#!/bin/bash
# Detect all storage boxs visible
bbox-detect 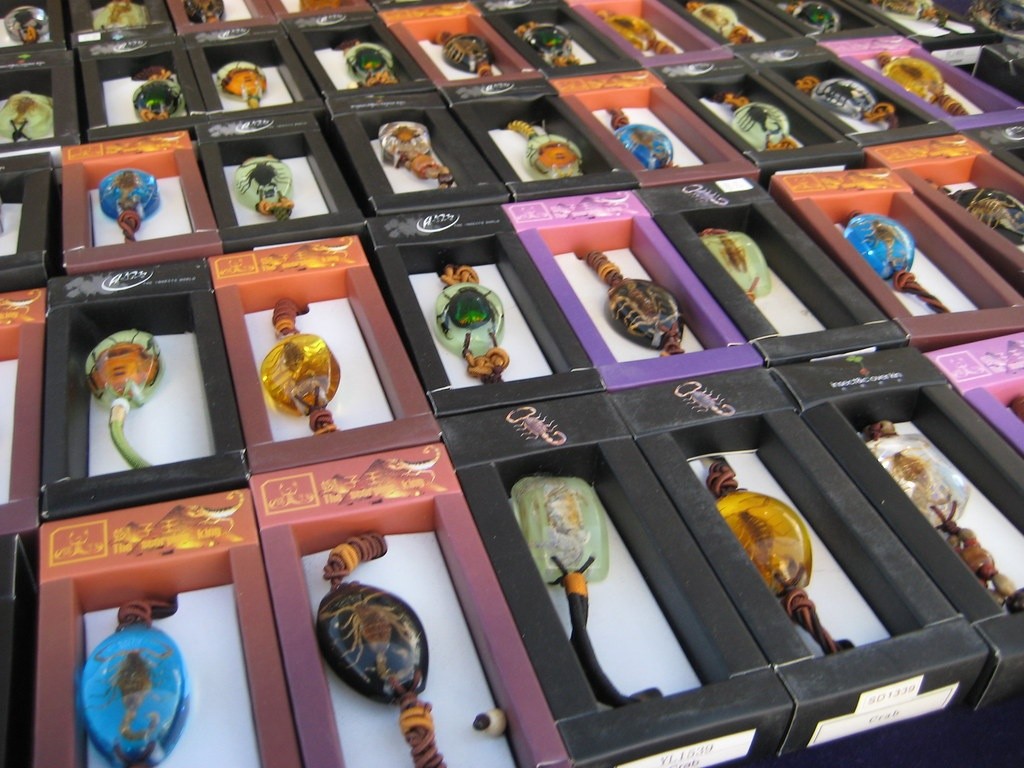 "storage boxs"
[0,0,1024,768]
